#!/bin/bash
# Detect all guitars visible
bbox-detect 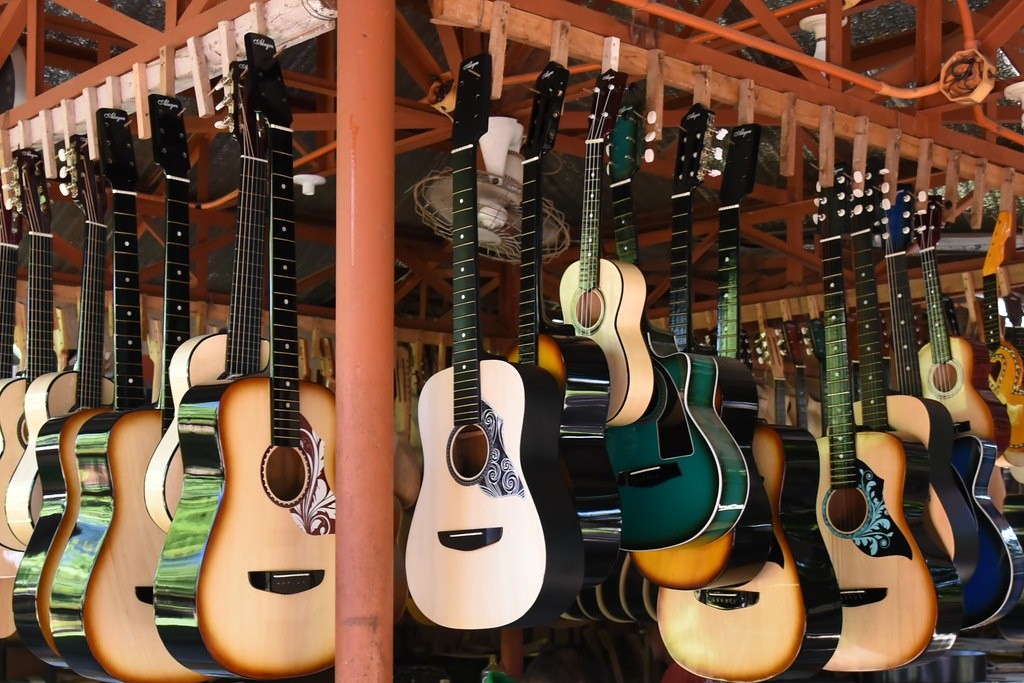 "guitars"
[153,33,339,682]
[557,64,656,428]
[402,50,590,635]
[603,101,1024,683]
[504,61,627,590]
[139,56,272,545]
[2,91,216,681]
